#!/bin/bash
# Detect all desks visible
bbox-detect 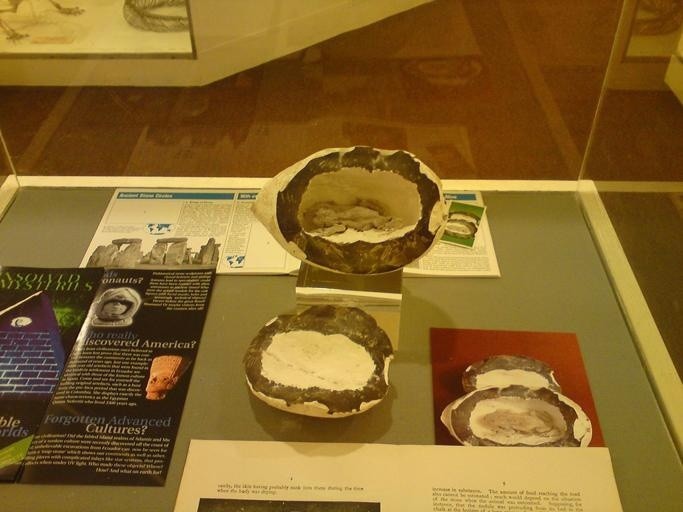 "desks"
[0,176,683,512]
